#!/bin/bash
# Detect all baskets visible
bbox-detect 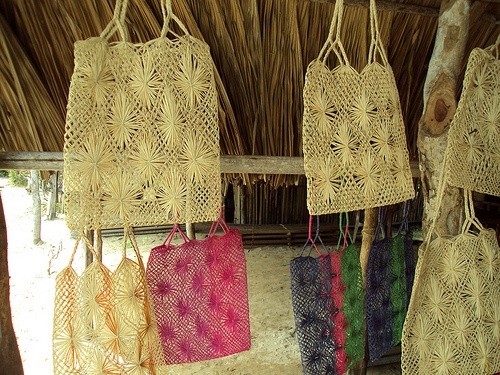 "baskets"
[442,30,500,199]
[145,222,252,365]
[300,0,415,216]
[62,0,223,231]
[399,145,500,374]
[357,201,418,364]
[51,232,168,375]
[289,214,367,374]
